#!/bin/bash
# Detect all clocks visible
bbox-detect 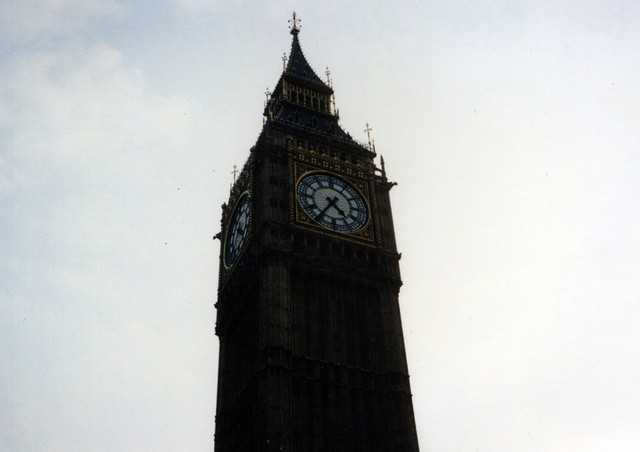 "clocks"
[221,189,253,270]
[295,167,372,236]
